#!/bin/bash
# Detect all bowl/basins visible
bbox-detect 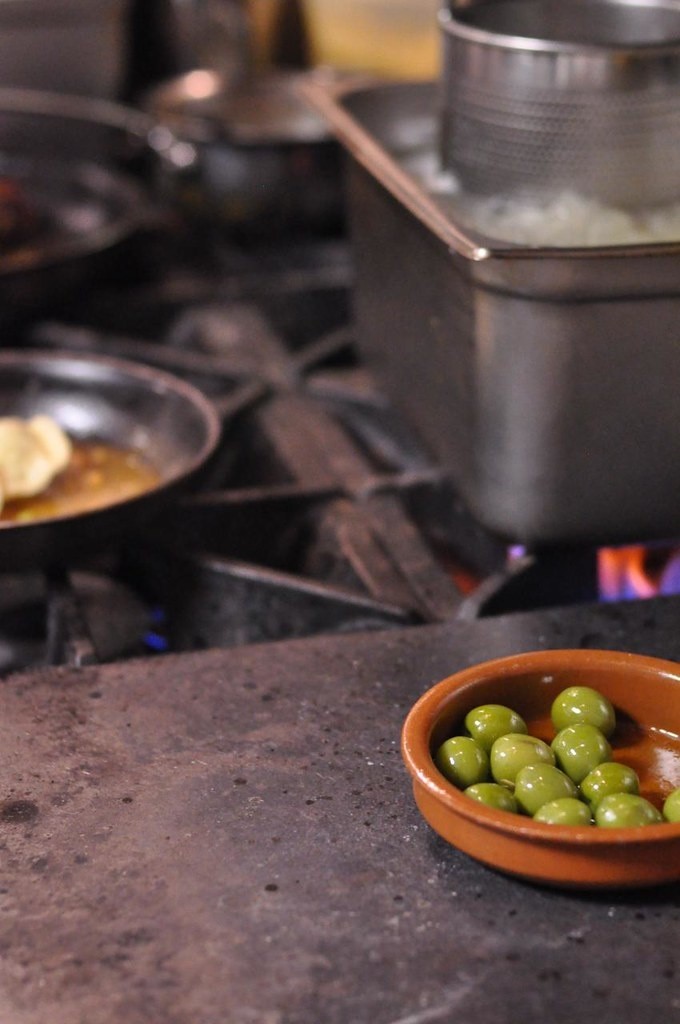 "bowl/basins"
[1,348,222,569]
[400,648,679,886]
